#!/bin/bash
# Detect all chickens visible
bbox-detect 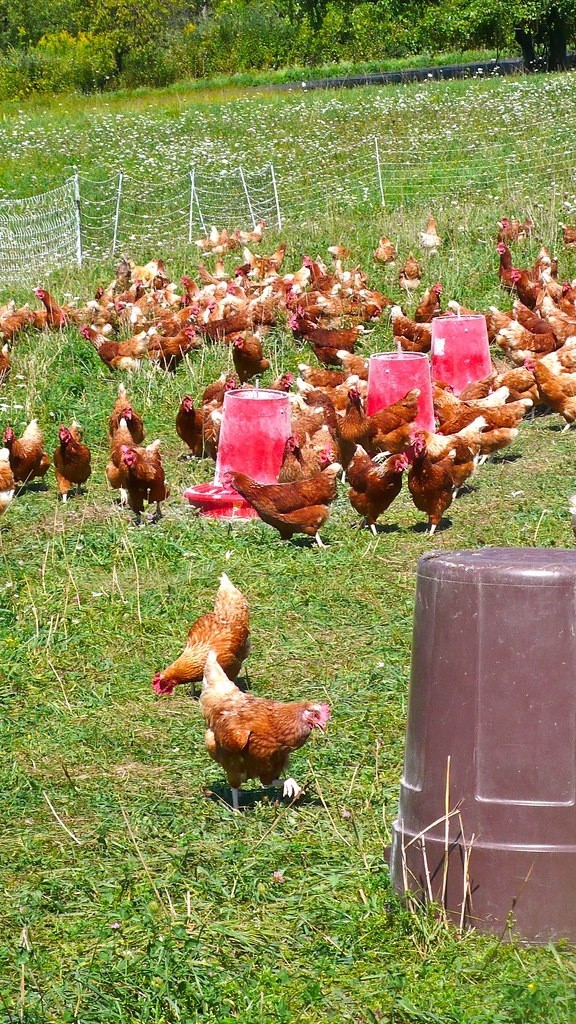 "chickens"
[0,215,575,549]
[198,650,330,811]
[150,572,251,697]
